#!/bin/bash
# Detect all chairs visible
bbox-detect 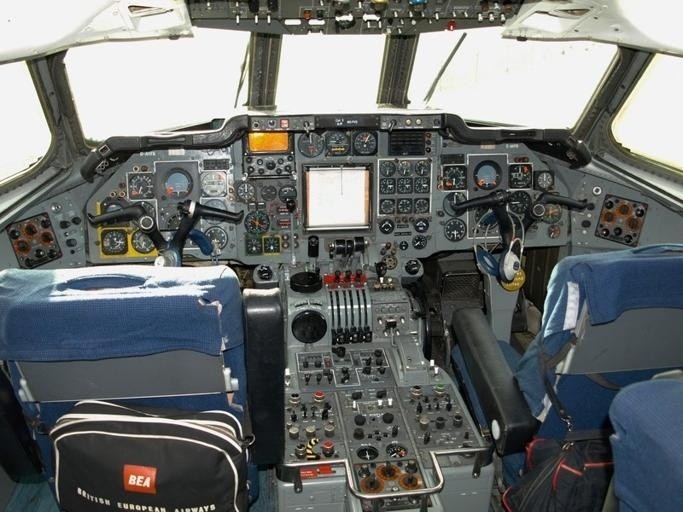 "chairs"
[0,263,260,506]
[451,242,682,512]
